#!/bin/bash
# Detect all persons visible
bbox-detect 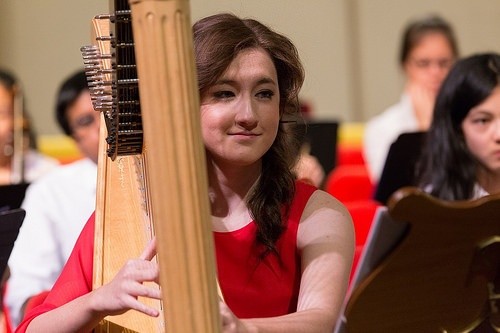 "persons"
[0,66,63,296]
[14,13,356,333]
[2,69,101,333]
[361,15,459,184]
[415,52,500,205]
